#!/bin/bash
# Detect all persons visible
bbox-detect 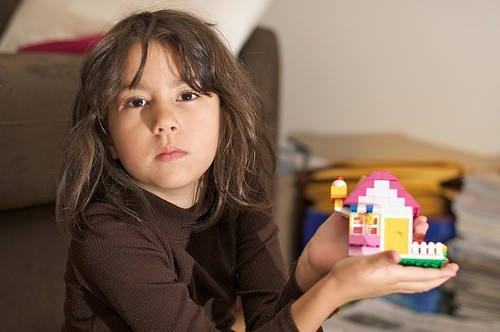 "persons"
[48,8,460,332]
